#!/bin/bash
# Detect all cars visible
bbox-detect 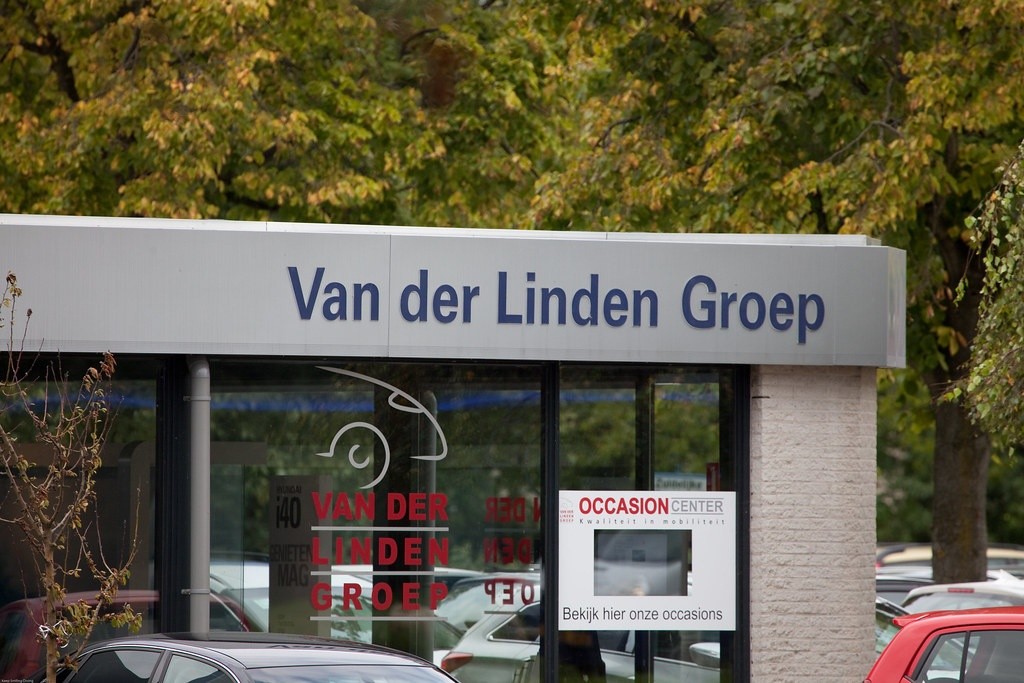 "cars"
[857,538,1024,683]
[14,632,460,683]
[0,555,722,683]
